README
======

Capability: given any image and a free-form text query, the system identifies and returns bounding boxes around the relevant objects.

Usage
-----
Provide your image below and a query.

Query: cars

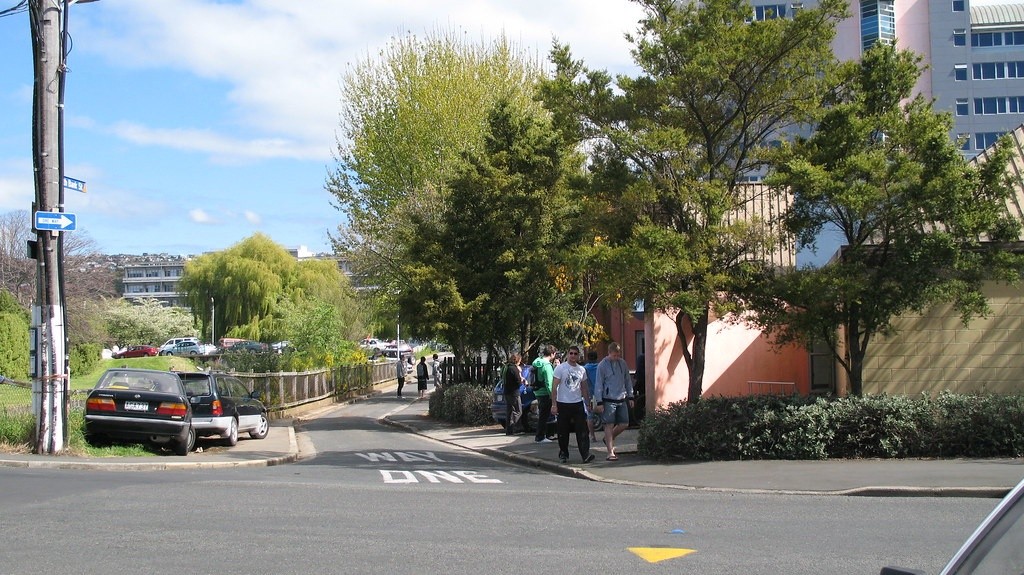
[491,364,640,433]
[111,344,158,358]
[84,368,196,456]
[356,337,453,373]
[880,479,1024,575]
[166,341,204,356]
[200,338,296,355]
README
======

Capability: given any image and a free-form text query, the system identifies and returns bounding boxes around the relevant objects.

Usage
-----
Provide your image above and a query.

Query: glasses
[570,352,578,356]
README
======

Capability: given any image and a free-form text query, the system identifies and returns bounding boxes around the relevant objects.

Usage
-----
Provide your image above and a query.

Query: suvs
[172,369,269,446]
[158,336,201,356]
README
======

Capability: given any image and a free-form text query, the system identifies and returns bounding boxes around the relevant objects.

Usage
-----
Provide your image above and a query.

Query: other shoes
[582,453,595,463]
[397,396,406,400]
[506,432,519,436]
[548,435,558,440]
[590,435,597,443]
[560,456,567,464]
[534,437,552,443]
[417,396,426,400]
[517,431,526,435]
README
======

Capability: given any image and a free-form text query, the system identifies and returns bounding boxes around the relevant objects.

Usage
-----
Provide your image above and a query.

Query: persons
[500,342,634,463]
[417,356,429,399]
[396,355,406,398]
[432,354,441,390]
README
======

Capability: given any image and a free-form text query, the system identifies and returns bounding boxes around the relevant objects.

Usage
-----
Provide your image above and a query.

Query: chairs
[112,382,129,388]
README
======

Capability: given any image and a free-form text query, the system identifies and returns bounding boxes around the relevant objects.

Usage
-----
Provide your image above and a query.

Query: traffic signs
[34,211,77,232]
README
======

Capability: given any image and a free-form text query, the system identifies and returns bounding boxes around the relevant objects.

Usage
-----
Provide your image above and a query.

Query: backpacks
[528,361,554,391]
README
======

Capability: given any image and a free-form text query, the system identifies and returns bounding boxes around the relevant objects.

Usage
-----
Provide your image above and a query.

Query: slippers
[605,454,618,461]
[603,437,617,454]
